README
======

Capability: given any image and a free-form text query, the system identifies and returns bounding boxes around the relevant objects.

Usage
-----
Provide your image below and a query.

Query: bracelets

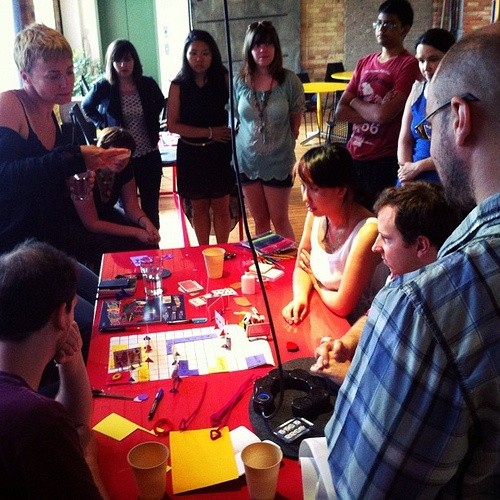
[207,125,217,140]
[137,214,148,224]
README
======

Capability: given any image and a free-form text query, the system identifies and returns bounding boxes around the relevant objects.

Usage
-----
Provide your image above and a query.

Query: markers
[148,389,163,419]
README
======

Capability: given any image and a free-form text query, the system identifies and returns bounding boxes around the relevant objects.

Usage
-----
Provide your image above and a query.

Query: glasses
[247,20,273,31]
[414,93,480,141]
[372,22,400,31]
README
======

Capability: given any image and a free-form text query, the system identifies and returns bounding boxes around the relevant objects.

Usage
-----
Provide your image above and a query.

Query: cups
[126,441,169,500]
[140,261,162,296]
[241,442,283,500]
[202,246,225,278]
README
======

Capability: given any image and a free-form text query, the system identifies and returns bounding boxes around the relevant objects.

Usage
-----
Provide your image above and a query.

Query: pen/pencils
[273,254,294,258]
[166,317,207,324]
[101,327,141,331]
[265,257,285,269]
[93,392,133,398]
[265,256,290,260]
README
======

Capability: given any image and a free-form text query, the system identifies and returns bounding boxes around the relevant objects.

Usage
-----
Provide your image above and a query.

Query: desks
[299,71,358,146]
[85,240,352,500]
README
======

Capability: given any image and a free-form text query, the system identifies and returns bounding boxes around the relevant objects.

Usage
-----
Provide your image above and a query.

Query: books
[98,294,187,330]
[98,277,136,297]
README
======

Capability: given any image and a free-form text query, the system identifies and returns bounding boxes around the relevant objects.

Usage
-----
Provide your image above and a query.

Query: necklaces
[250,74,276,133]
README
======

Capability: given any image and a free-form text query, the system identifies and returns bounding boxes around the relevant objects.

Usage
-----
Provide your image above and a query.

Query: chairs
[296,70,320,137]
[323,62,345,118]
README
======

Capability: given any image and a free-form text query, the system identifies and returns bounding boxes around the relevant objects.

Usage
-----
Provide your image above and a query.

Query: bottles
[241,268,255,295]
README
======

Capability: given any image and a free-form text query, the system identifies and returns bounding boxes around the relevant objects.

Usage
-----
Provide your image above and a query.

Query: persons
[82,38,168,232]
[0,238,92,500]
[394,27,456,188]
[309,178,457,378]
[279,144,388,327]
[224,21,306,240]
[329,0,417,166]
[0,23,129,331]
[323,23,500,499]
[68,127,161,254]
[165,29,233,247]
